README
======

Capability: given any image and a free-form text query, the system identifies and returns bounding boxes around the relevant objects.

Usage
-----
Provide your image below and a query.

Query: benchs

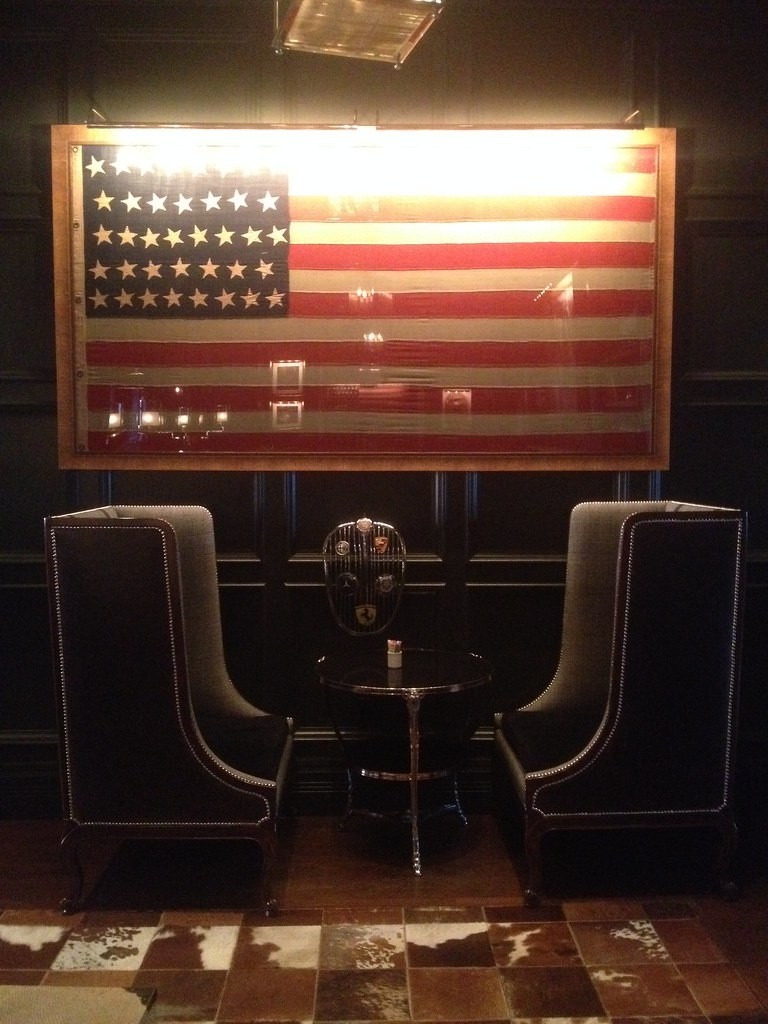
[43,502,294,917]
[484,498,749,907]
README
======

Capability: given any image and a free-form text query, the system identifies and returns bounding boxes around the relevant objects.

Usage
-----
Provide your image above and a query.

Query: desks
[317,650,492,878]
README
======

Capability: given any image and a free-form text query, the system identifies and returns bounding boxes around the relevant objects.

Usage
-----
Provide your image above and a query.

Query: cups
[387,651,402,668]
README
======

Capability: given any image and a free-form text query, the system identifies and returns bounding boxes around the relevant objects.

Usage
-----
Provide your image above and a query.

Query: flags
[69,144,655,460]
